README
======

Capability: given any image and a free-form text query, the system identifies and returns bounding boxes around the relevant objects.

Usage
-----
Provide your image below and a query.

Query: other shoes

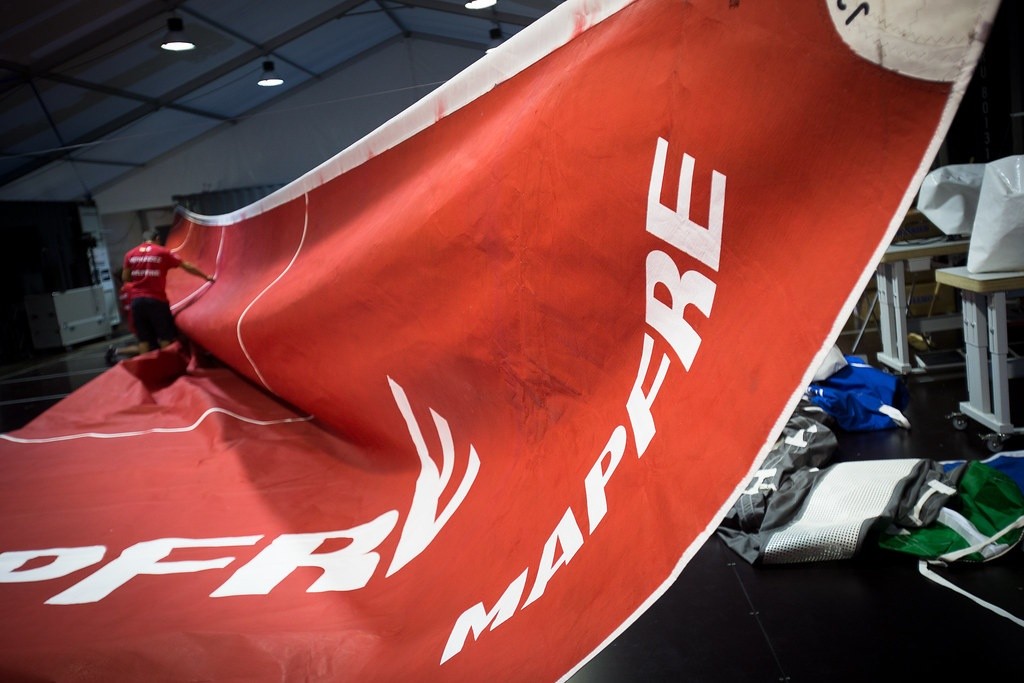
[105,344,116,368]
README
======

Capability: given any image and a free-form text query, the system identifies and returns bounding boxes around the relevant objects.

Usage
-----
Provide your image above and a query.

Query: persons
[106,231,216,366]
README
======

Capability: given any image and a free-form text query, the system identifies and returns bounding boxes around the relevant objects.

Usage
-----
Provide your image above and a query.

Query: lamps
[257,53,284,86]
[160,10,195,51]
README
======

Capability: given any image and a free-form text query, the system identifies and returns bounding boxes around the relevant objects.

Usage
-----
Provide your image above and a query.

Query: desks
[873,238,1024,452]
